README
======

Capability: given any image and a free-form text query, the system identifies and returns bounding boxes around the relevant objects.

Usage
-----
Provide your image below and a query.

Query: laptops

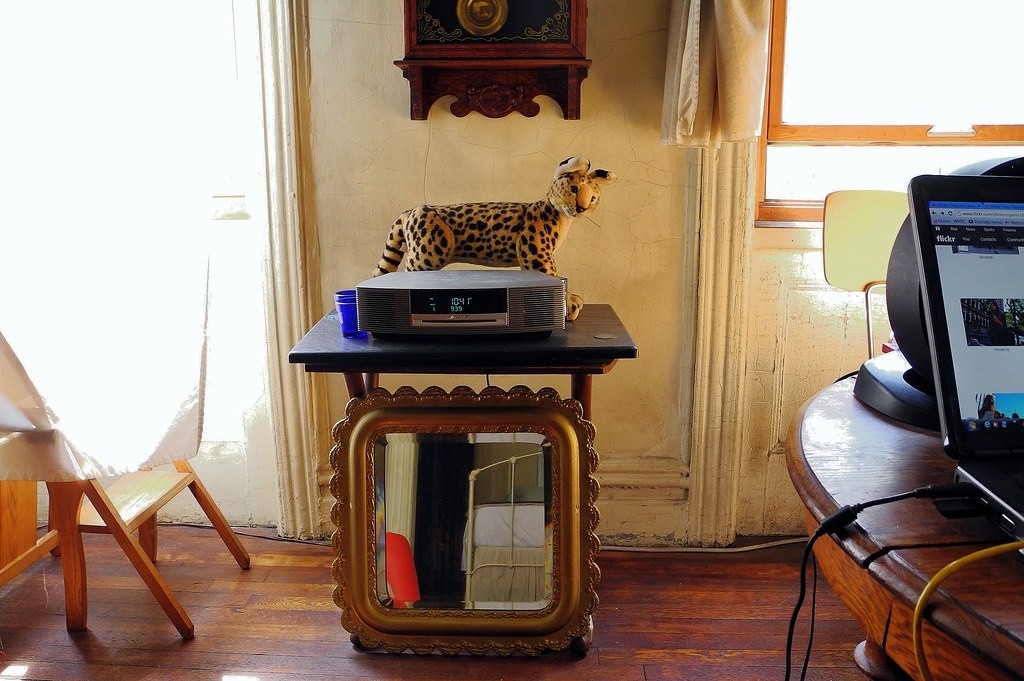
[909,175,1024,540]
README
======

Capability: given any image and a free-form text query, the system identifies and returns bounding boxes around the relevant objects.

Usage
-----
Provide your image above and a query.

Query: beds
[461,452,548,608]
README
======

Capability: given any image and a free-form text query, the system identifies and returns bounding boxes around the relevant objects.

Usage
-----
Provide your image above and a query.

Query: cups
[335,290,367,339]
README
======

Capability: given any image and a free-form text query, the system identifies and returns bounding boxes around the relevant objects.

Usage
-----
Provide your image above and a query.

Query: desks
[785,372,1024,680]
[289,302,637,656]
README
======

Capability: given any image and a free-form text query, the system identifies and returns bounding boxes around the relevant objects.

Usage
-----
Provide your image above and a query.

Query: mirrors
[328,386,600,654]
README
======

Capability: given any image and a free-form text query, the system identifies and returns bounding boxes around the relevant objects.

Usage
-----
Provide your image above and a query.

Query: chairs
[822,190,910,359]
